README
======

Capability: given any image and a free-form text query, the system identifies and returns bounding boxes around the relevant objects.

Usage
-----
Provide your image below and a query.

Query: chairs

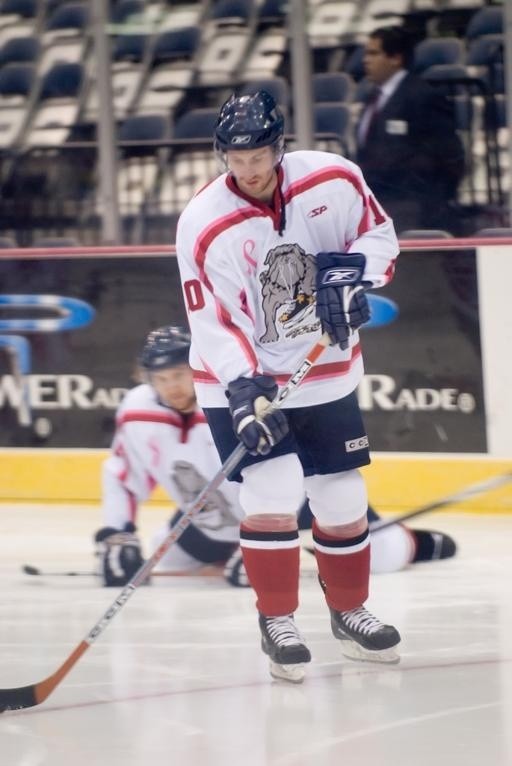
[0,0,511,247]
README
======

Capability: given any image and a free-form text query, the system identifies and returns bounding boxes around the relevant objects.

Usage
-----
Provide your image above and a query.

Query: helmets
[213,90,286,177]
[140,321,197,412]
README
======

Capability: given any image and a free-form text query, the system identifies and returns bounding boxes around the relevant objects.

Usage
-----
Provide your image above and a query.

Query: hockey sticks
[23,565,232,578]
[1,329,332,711]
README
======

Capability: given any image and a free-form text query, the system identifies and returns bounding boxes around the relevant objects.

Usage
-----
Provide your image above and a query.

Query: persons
[176,88,401,669]
[96,326,458,587]
[352,24,464,234]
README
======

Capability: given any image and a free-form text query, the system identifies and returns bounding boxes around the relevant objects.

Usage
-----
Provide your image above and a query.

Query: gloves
[95,523,146,590]
[222,373,292,460]
[313,252,372,350]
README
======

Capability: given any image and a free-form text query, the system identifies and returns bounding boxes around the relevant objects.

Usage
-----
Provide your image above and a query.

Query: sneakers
[408,527,459,564]
[330,603,403,652]
[258,613,313,668]
